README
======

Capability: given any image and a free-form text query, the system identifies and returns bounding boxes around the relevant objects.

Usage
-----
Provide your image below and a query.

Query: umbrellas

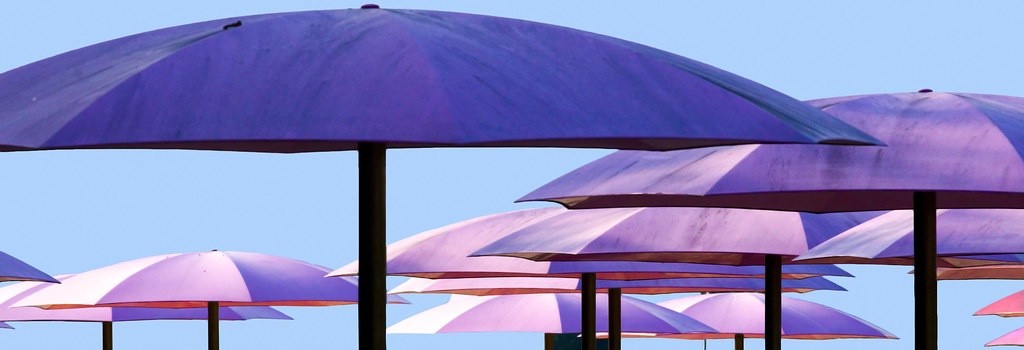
[0,5,1024,350]
